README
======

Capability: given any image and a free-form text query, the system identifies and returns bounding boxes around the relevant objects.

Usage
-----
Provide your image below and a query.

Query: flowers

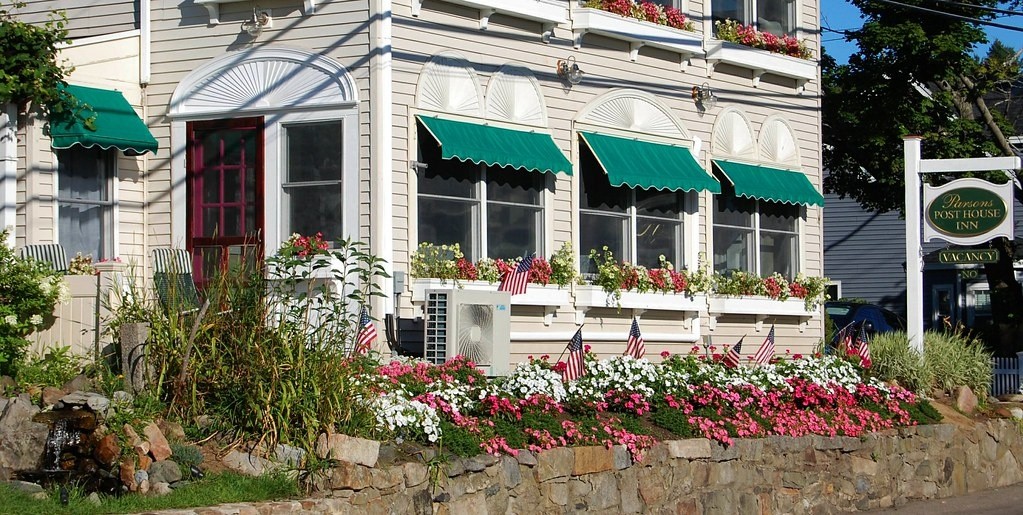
[279,230,330,258]
[65,252,123,276]
[588,247,710,314]
[712,268,831,311]
[410,240,576,288]
[584,0,696,32]
[714,17,813,61]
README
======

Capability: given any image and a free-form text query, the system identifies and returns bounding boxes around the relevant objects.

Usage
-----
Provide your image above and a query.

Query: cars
[824,302,906,355]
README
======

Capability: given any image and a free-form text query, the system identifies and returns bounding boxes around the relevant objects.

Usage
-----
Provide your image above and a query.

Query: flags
[755,325,775,366]
[562,328,586,384]
[354,307,377,354]
[840,323,853,349]
[854,326,872,366]
[627,319,645,359]
[723,337,743,369]
[500,255,532,296]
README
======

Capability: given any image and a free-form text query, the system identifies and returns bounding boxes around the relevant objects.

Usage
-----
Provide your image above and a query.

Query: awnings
[51,83,158,155]
[714,160,825,207]
[416,114,572,176]
[578,131,721,194]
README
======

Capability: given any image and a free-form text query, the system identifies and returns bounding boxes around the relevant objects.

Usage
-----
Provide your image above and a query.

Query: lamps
[557,55,585,84]
[692,82,719,110]
[246,4,272,37]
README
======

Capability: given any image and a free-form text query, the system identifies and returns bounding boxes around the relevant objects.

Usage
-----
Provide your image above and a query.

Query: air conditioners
[424,289,511,377]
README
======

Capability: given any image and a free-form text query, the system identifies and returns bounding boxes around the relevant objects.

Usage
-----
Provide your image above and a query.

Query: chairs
[151,248,233,329]
